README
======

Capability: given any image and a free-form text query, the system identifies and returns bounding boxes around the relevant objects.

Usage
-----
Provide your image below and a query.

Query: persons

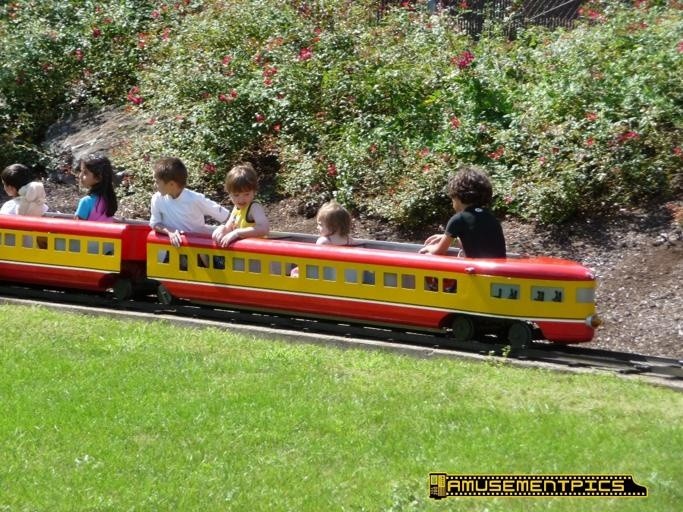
[148,155,232,270]
[212,164,269,272]
[74,155,118,223]
[0,164,49,218]
[290,200,360,283]
[418,165,507,293]
